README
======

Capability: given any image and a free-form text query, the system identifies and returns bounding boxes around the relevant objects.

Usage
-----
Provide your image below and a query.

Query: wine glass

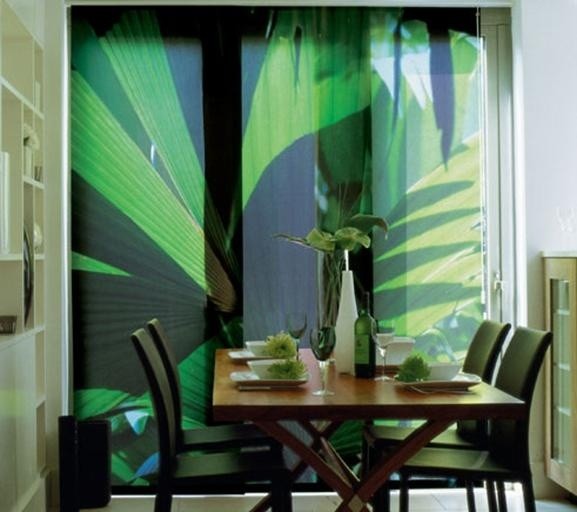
[285,310,308,362]
[309,326,336,396]
[371,317,396,383]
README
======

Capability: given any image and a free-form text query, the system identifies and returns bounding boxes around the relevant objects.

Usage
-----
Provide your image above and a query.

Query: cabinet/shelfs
[542,257,577,496]
[0,1,48,511]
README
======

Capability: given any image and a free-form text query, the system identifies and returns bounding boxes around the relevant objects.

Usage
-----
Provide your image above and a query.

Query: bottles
[352,290,377,380]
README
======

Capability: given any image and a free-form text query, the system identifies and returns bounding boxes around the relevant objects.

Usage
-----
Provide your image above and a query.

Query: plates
[227,350,278,362]
[392,372,483,387]
[374,354,418,372]
[232,370,309,386]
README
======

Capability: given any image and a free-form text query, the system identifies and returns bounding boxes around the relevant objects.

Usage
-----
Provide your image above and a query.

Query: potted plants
[271,207,390,327]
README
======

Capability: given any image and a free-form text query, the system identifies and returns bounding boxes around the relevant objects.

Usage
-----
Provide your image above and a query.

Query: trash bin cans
[79,420,111,507]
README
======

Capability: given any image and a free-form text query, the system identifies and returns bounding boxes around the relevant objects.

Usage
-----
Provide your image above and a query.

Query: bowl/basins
[246,358,290,377]
[243,340,271,354]
[422,359,462,379]
[376,335,416,354]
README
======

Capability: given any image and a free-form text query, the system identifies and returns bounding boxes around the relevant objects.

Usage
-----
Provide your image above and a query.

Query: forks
[409,386,476,395]
